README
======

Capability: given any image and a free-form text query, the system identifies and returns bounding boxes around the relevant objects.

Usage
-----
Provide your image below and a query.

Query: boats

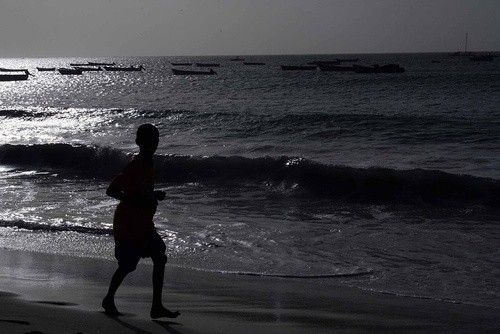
[468,52,493,63]
[281,64,317,72]
[171,67,218,77]
[243,62,265,66]
[232,57,245,62]
[308,58,405,77]
[171,62,193,66]
[0,57,144,86]
[196,62,221,68]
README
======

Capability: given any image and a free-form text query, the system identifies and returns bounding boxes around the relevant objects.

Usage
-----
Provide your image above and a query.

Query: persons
[101,124,181,319]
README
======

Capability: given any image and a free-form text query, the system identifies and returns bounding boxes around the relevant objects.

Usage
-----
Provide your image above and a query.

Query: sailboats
[449,33,474,57]
